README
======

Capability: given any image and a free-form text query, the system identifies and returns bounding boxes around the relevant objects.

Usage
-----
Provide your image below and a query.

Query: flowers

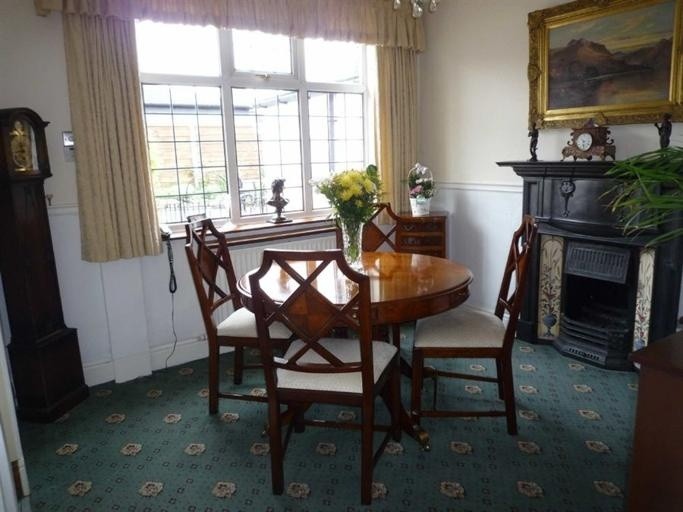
[405,163,438,201]
[310,163,384,219]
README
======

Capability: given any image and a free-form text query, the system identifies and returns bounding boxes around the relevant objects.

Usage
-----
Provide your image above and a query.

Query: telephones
[159,224,172,234]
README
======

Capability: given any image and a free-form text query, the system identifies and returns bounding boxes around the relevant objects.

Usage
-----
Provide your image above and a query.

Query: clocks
[0,109,90,425]
[561,129,615,160]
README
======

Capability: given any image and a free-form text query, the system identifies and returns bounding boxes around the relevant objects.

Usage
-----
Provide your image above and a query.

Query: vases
[410,195,430,215]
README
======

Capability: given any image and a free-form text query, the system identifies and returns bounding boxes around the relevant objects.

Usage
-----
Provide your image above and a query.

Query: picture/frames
[525,2,682,127]
